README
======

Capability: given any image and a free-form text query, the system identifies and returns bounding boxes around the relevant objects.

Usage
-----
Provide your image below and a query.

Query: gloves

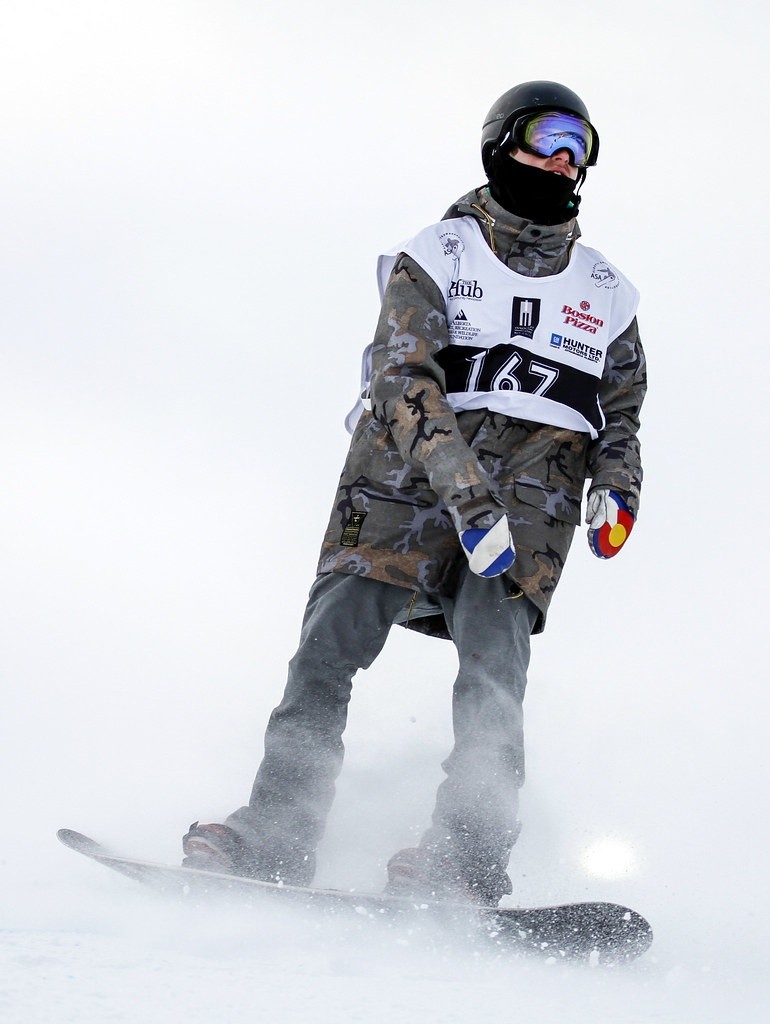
[459,513,516,578]
[585,489,635,560]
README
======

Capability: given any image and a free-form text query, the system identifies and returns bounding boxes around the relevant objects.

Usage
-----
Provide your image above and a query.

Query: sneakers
[183,820,316,888]
[382,848,502,908]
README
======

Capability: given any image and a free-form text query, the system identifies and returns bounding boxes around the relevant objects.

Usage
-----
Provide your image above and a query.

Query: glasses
[511,108,596,167]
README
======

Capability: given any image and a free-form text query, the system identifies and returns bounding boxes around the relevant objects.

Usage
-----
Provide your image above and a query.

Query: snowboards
[55,828,655,960]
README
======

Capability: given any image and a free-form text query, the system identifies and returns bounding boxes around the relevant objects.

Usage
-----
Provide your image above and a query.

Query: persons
[182,82,648,905]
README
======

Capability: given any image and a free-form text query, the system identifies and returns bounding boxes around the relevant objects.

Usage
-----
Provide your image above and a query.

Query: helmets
[481,81,600,177]
